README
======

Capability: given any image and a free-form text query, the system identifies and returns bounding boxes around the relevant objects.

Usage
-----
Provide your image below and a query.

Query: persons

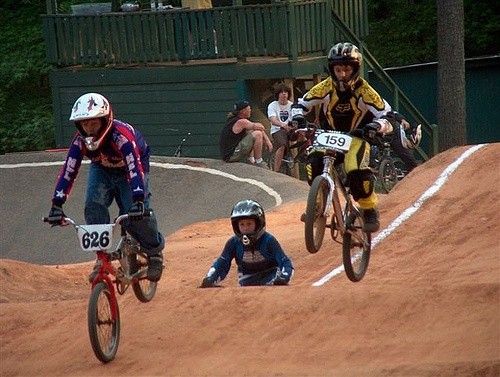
[268,84,299,180]
[202,199,293,286]
[48,92,165,282]
[377,110,417,174]
[288,41,396,233]
[219,99,274,169]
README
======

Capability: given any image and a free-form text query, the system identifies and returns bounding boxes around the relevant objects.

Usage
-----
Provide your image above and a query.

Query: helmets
[327,42,363,93]
[68,92,114,152]
[230,198,267,247]
[398,123,422,150]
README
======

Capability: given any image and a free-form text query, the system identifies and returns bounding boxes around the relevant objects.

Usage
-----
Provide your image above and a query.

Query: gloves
[201,277,214,287]
[291,114,306,129]
[268,277,287,285]
[363,121,381,138]
[124,194,146,222]
[48,199,69,225]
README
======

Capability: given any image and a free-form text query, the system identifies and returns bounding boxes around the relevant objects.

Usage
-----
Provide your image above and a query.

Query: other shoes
[147,252,164,282]
[361,208,379,233]
[248,159,256,165]
[255,161,270,170]
[300,202,320,223]
[89,259,110,282]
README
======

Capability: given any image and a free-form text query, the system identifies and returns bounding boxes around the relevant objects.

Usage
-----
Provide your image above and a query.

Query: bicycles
[42,207,164,363]
[289,127,371,283]
[172,113,412,194]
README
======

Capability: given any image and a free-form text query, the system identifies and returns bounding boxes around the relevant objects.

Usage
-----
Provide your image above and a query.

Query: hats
[233,101,249,111]
